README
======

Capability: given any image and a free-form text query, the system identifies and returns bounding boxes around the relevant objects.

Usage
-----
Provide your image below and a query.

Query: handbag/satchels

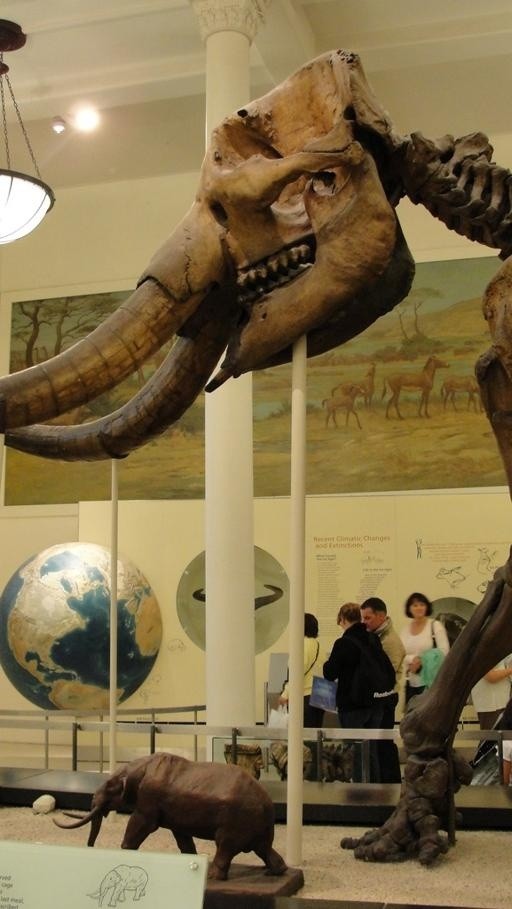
[309,676,338,714]
[266,709,289,729]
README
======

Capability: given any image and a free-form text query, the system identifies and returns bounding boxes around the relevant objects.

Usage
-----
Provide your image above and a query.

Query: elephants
[53,752,286,881]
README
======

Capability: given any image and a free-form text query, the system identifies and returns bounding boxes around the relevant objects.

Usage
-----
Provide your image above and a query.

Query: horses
[322,384,365,430]
[381,355,450,420]
[331,362,376,413]
[440,374,486,414]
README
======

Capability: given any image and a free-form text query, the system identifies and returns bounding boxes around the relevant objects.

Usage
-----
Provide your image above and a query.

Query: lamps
[0,19,54,246]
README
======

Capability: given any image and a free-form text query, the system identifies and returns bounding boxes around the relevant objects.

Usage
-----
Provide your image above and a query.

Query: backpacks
[343,635,399,706]
